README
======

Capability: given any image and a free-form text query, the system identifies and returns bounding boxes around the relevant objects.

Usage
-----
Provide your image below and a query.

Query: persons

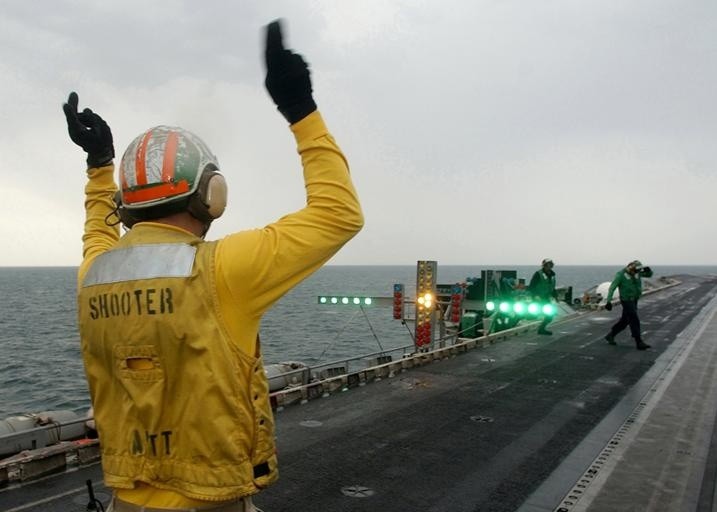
[600,260,655,350]
[508,275,516,286]
[527,258,559,335]
[59,16,365,511]
[501,274,514,295]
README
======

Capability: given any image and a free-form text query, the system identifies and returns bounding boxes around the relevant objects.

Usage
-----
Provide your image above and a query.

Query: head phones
[113,170,227,229]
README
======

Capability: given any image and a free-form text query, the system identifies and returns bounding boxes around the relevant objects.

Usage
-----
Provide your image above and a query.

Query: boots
[537,323,552,335]
[604,329,618,346]
[634,335,652,350]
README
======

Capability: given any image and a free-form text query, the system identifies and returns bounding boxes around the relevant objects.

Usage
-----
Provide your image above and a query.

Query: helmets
[116,123,223,210]
[541,257,554,265]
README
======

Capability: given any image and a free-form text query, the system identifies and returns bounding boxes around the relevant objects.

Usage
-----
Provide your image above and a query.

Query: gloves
[603,301,613,312]
[261,18,318,126]
[60,89,116,169]
